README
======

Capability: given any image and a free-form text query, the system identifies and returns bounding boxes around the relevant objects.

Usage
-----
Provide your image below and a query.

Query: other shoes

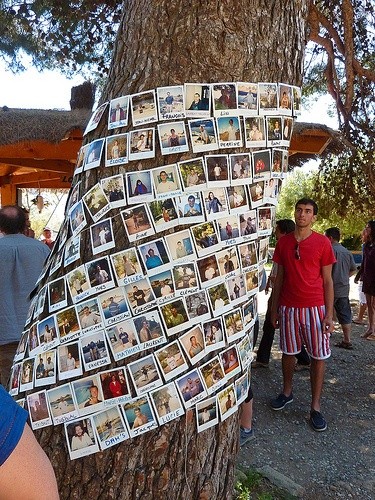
[366,334,375,340]
[294,364,311,371]
[251,361,269,368]
[352,319,364,324]
[360,331,373,338]
[239,426,254,446]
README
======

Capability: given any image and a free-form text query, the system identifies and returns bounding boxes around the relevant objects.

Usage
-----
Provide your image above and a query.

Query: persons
[270,198,338,432]
[239,269,267,446]
[352,220,375,341]
[12,80,302,452]
[1,204,52,390]
[0,385,61,500]
[324,227,357,350]
[252,219,311,371]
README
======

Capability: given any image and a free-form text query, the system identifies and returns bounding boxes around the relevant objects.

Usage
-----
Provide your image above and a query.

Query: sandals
[335,340,354,349]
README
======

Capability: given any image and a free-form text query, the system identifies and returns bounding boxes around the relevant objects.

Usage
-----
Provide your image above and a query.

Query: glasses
[295,245,301,260]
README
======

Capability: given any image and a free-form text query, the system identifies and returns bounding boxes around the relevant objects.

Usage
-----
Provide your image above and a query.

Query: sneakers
[310,408,327,432]
[270,392,293,411]
